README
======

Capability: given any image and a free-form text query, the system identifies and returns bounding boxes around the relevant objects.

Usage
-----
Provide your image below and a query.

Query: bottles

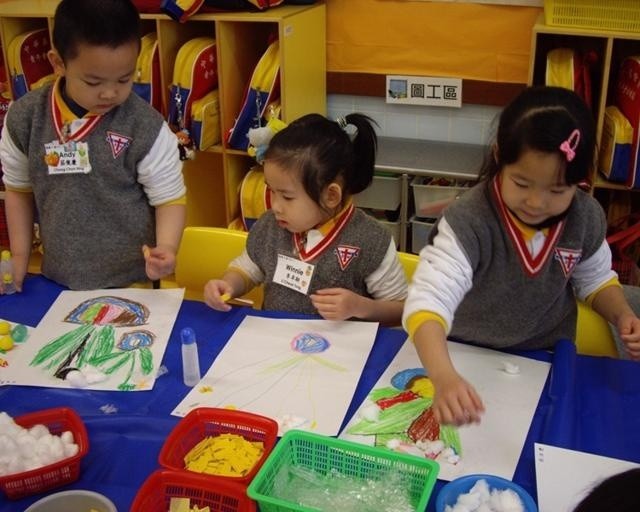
[180,327,202,387]
[0,250,17,295]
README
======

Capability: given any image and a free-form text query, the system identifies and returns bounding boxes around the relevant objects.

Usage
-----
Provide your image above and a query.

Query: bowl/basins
[24,489,119,511]
[435,473,536,511]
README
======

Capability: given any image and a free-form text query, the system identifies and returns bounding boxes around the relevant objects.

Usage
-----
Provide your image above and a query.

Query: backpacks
[599,57,640,189]
[168,38,220,161]
[240,166,271,231]
[225,40,280,151]
[6,28,56,101]
[546,48,597,112]
[132,33,164,117]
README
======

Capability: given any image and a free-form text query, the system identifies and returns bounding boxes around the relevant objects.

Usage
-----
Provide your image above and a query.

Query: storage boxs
[351,173,402,211]
[409,175,482,219]
[367,214,401,250]
[409,216,438,255]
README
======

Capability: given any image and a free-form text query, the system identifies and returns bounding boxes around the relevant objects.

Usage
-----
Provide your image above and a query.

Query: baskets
[0,406,90,499]
[158,407,278,483]
[247,430,440,512]
[129,470,256,511]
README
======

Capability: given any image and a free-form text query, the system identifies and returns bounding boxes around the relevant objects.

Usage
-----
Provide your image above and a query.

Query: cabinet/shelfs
[526,9,638,287]
[328,132,498,257]
[0,1,328,234]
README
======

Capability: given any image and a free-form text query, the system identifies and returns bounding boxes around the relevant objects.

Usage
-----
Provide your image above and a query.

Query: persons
[203,112,408,321]
[0,0,189,296]
[401,85,640,428]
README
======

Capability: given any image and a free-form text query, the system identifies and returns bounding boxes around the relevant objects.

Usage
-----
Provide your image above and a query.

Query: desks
[1,270,640,512]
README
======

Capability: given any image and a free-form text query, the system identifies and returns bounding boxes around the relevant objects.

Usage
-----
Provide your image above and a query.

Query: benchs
[175,226,622,360]
